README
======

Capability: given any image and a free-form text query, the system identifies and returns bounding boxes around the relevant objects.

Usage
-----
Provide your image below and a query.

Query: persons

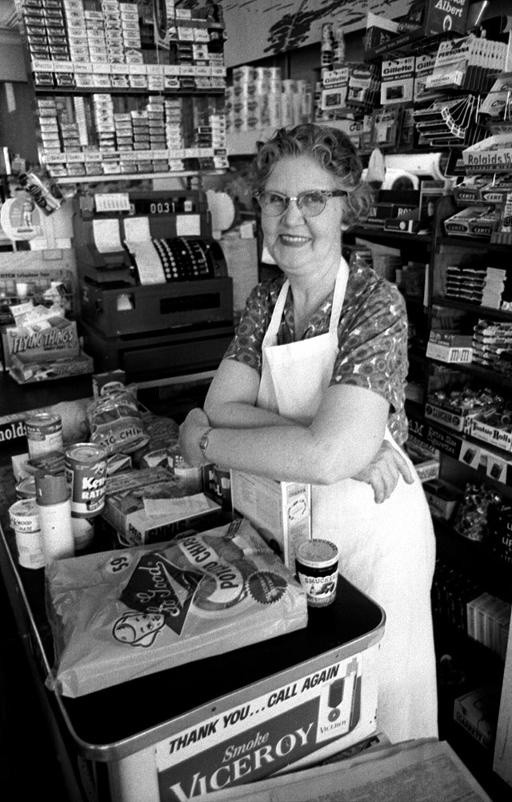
[178,122,443,747]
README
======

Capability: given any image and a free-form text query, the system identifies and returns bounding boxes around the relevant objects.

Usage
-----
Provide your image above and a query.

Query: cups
[249,186,352,218]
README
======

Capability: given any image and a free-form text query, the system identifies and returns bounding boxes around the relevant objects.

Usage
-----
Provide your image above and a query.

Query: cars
[17,282,35,305]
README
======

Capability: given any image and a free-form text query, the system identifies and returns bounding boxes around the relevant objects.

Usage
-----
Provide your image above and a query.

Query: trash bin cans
[199,427,219,467]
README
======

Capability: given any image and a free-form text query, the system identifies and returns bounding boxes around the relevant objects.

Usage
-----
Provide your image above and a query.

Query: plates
[347,188,512,736]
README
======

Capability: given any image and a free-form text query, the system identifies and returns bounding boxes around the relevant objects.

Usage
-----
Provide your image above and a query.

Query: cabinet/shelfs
[34,472,77,566]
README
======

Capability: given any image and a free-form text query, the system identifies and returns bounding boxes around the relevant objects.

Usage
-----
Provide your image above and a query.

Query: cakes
[295,539,339,609]
[25,412,64,472]
[65,443,107,519]
[224,65,307,128]
